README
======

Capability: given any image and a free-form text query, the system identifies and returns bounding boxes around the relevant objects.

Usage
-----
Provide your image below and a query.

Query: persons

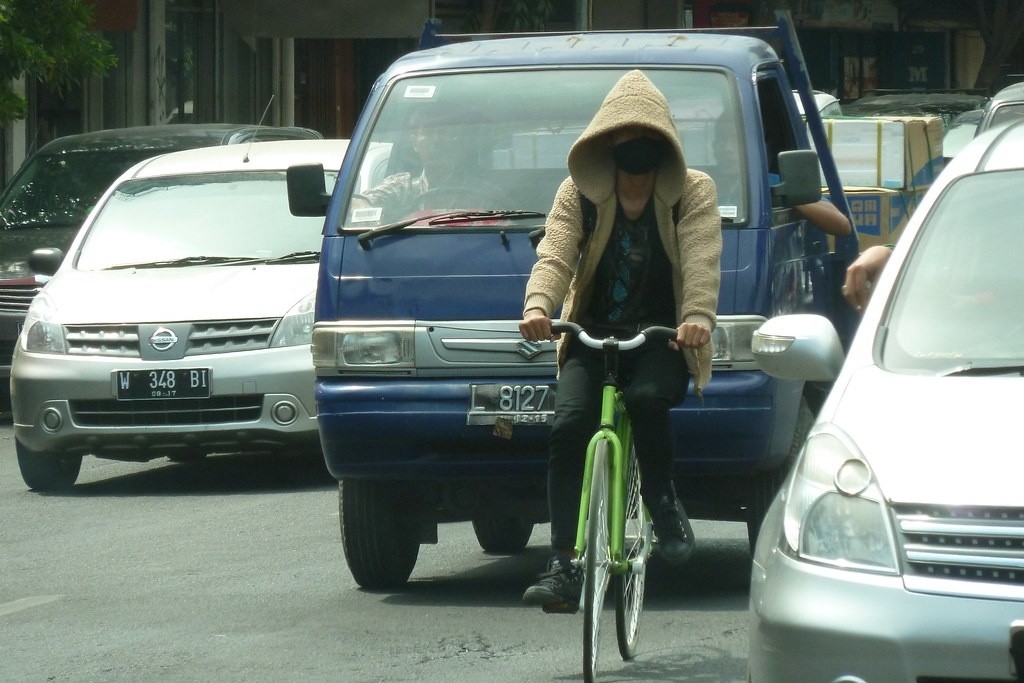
[356,99,521,225]
[517,68,723,608]
[842,243,897,316]
[708,113,852,236]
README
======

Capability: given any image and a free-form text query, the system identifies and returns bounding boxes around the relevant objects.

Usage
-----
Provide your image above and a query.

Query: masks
[612,137,663,175]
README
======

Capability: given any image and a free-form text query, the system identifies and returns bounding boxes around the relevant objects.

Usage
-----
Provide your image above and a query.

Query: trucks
[285,6,866,589]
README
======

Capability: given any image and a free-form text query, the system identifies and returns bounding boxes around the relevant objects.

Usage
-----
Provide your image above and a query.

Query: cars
[1,125,326,428]
[9,138,397,491]
[743,112,1024,683]
[785,81,1024,163]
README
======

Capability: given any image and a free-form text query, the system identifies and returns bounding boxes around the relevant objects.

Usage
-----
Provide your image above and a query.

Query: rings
[848,295,883,297]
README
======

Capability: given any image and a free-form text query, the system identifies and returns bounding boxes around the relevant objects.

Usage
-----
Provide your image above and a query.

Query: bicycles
[541,320,703,683]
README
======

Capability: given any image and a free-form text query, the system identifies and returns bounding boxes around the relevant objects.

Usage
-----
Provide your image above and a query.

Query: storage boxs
[804,116,946,254]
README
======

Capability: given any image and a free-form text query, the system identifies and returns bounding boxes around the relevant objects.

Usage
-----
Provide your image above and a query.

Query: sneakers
[522,552,584,611]
[642,487,695,567]
